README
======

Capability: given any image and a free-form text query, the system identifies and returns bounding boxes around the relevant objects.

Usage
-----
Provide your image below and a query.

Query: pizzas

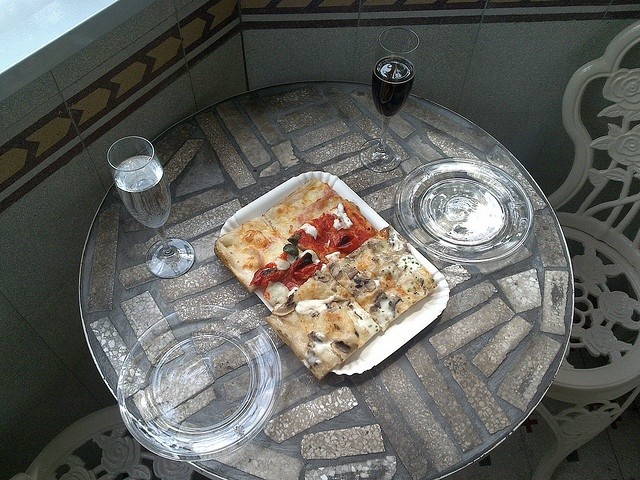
[214,177,378,311]
[265,271,379,382]
[329,224,438,333]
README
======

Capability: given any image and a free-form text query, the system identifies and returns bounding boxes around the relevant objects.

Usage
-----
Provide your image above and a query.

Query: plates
[116,305,283,463]
[218,171,450,376]
[395,157,535,263]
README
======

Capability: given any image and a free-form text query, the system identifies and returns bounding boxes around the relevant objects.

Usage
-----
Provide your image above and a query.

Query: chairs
[530,20,640,480]
[9,404,202,480]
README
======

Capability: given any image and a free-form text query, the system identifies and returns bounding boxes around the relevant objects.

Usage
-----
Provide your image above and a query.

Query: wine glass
[359,27,420,174]
[107,135,196,279]
